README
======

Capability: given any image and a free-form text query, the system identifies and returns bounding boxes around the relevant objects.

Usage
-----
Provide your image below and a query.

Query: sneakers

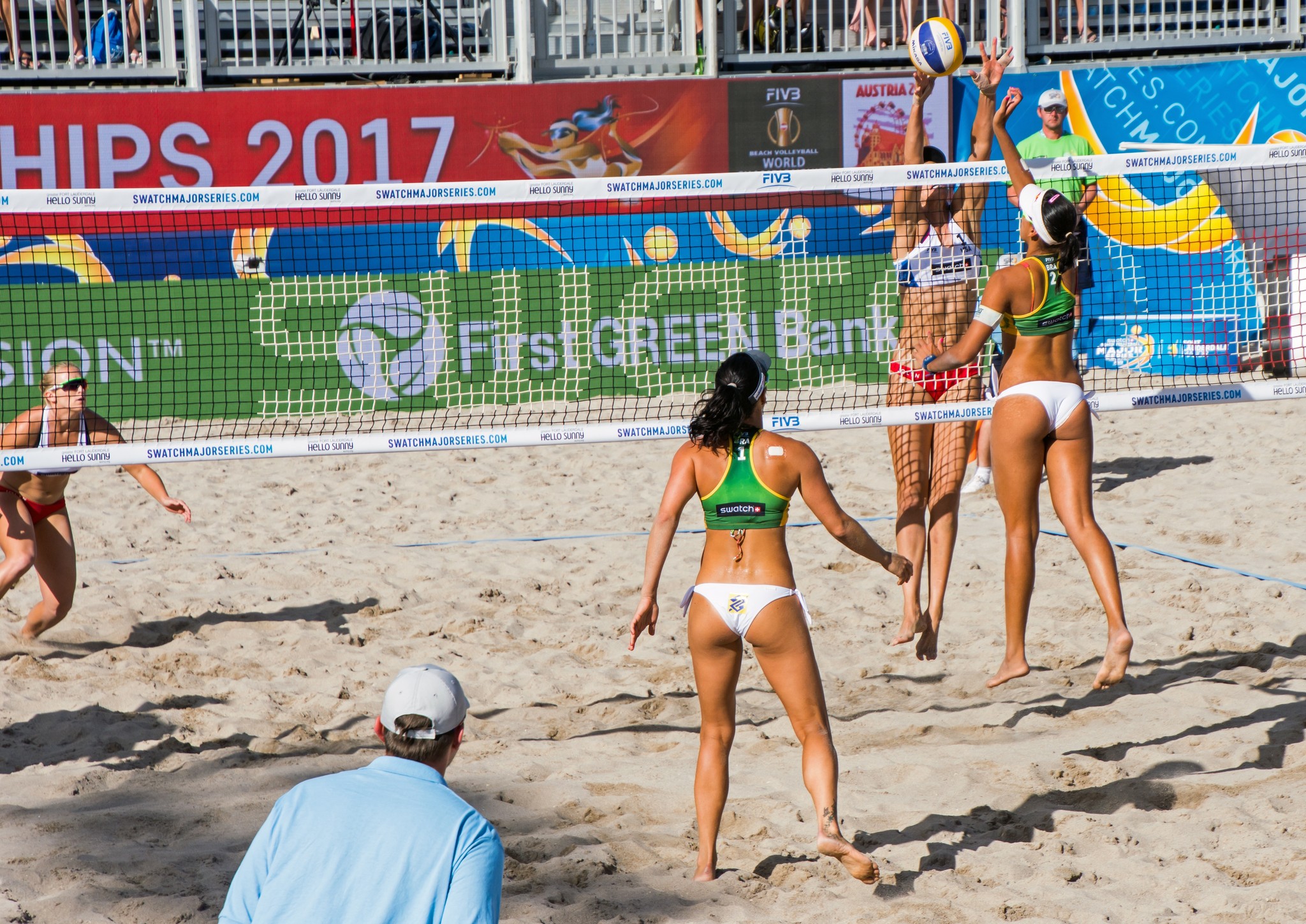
[740,29,763,50]
[693,36,703,74]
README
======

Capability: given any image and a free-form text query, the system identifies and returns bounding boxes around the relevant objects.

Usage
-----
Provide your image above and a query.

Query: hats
[380,664,471,742]
[923,145,947,163]
[1039,88,1068,108]
[1019,183,1077,246]
[731,349,772,403]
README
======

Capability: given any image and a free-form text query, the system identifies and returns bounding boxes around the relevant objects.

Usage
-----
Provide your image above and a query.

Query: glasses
[47,377,88,391]
[1040,105,1065,113]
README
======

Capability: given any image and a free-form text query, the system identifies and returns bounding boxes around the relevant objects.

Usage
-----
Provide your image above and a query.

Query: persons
[886,38,1014,654]
[218,663,503,924]
[0,0,1097,87]
[628,350,914,883]
[0,361,192,638]
[960,88,1105,495]
[912,77,1132,692]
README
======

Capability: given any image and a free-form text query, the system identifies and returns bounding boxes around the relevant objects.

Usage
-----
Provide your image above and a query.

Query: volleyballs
[907,17,967,77]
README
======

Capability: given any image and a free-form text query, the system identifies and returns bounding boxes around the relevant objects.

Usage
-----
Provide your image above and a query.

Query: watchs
[923,355,940,375]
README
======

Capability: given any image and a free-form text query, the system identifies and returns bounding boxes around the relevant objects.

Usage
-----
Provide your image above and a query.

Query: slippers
[1079,28,1097,42]
[128,52,152,64]
[11,56,41,68]
[1047,30,1068,41]
[888,37,904,45]
[66,53,85,64]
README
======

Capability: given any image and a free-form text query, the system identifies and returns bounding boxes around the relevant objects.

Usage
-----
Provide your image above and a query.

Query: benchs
[0,0,1306,85]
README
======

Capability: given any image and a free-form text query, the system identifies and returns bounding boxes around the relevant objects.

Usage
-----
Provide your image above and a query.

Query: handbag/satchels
[756,5,826,52]
[84,9,124,63]
